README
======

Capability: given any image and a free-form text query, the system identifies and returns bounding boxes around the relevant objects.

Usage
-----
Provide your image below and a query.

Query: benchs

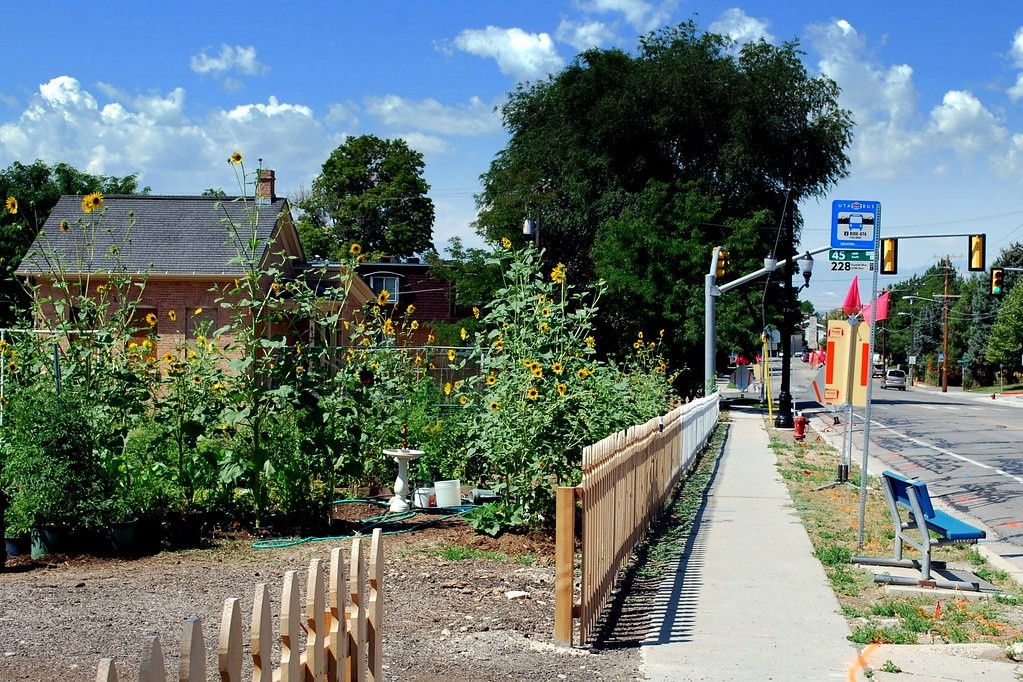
[879,471,987,581]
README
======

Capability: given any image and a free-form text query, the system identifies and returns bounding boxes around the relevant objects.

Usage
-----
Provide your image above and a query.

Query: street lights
[897,312,914,386]
[764,185,815,431]
[901,295,948,393]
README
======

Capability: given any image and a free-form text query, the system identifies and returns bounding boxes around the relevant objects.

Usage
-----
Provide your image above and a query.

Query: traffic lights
[990,267,1005,298]
[713,246,731,278]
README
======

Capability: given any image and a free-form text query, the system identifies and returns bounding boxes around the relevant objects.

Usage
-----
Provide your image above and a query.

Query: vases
[3,494,330,563]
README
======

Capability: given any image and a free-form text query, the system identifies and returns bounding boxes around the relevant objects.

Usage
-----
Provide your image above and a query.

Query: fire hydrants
[793,416,808,442]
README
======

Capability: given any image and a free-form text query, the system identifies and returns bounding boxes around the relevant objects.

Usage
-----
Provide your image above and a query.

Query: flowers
[1,148,285,509]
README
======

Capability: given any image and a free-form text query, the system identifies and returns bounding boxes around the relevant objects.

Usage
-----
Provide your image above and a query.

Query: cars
[880,369,907,390]
[794,350,811,364]
[872,361,887,378]
